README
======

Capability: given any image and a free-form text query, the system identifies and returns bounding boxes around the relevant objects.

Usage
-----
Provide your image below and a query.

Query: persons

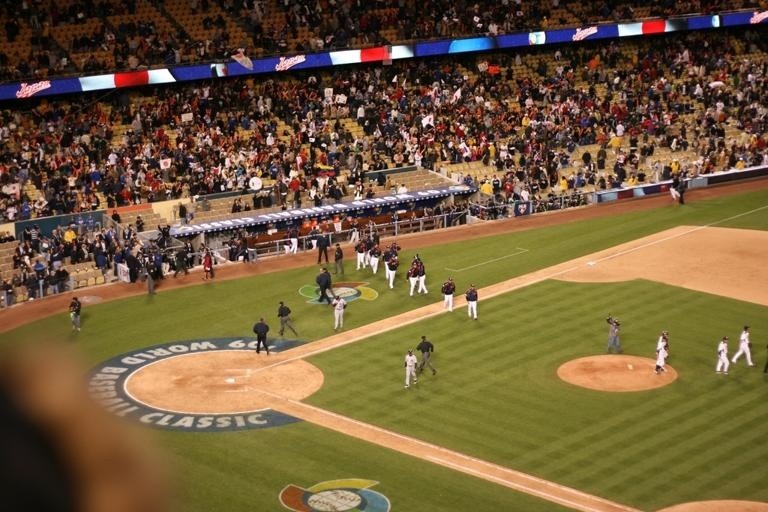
[654,343,669,374]
[0,1,765,304]
[403,348,419,389]
[441,277,456,314]
[607,314,625,355]
[69,297,83,331]
[731,324,756,368]
[464,283,478,321]
[277,301,299,337]
[714,335,730,375]
[416,335,438,375]
[656,330,670,358]
[252,317,269,351]
[332,295,347,329]
